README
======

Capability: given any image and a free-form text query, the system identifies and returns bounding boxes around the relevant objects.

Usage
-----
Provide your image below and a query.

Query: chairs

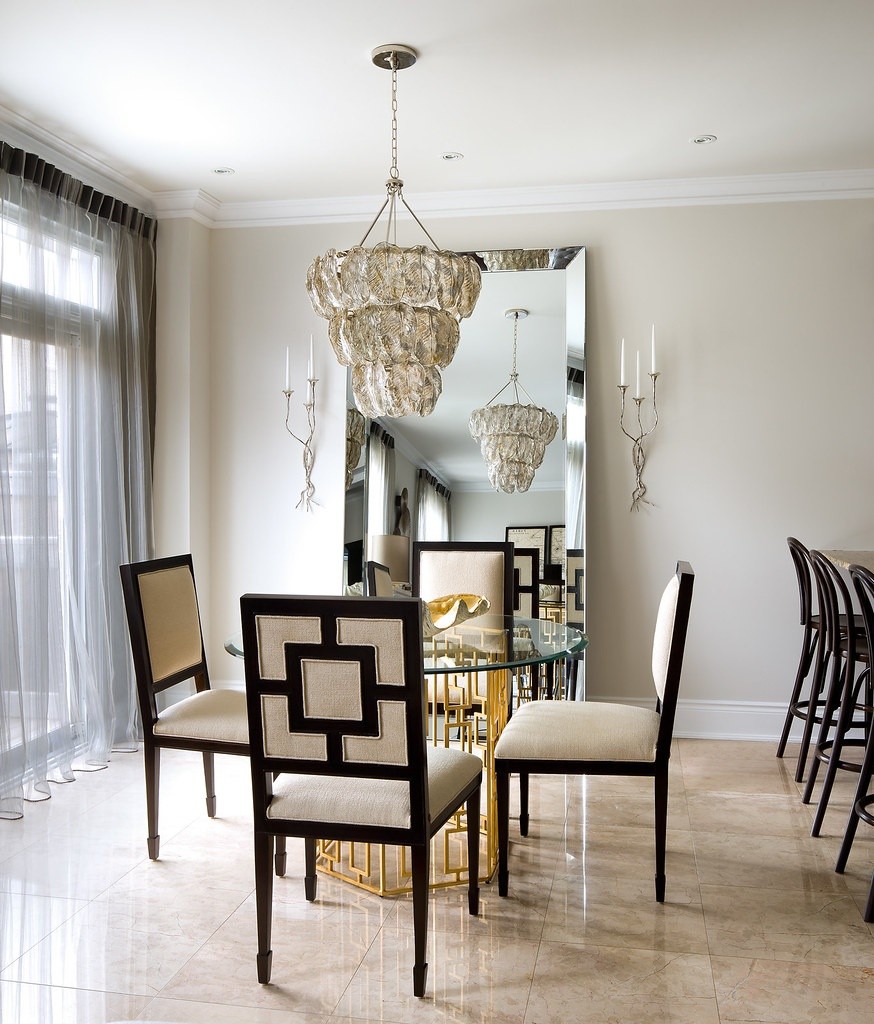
[412,539,515,744]
[236,589,484,999]
[119,553,289,879]
[773,534,874,924]
[537,580,562,604]
[513,547,541,620]
[491,559,696,902]
[367,561,395,599]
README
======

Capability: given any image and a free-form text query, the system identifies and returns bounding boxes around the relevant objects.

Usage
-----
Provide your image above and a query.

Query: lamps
[468,307,560,496]
[303,44,485,423]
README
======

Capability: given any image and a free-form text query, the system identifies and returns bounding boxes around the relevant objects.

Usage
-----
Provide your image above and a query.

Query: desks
[538,604,566,623]
[818,548,874,576]
[221,612,590,902]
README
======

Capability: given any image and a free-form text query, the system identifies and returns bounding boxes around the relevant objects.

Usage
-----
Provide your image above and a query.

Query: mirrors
[338,244,590,702]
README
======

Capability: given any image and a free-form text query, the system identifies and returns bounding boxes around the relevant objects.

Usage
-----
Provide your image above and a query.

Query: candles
[637,349,640,397]
[310,333,315,378]
[286,346,291,390]
[651,323,656,374]
[620,338,625,385]
[307,360,311,402]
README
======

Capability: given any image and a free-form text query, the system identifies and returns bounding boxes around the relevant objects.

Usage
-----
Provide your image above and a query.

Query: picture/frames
[548,524,567,584]
[506,526,547,583]
